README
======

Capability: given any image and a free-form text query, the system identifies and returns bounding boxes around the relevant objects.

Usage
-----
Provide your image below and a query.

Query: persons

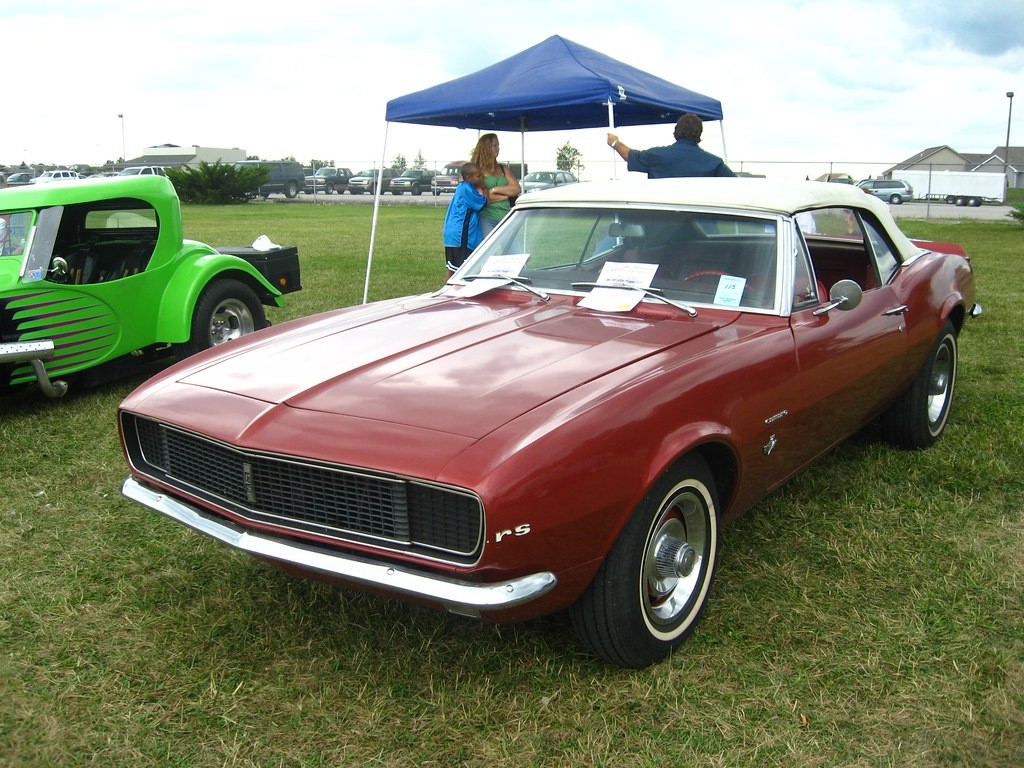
[472,133,521,265]
[608,114,737,235]
[444,163,489,286]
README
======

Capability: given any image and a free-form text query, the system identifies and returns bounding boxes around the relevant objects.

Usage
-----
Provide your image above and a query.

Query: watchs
[611,139,619,147]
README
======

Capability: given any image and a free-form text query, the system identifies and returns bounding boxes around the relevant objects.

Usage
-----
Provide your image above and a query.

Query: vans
[0,165,174,184]
[227,160,306,198]
[857,180,913,205]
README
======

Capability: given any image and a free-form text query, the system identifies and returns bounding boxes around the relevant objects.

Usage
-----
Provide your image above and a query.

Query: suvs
[347,168,400,196]
[302,168,354,196]
[389,168,442,196]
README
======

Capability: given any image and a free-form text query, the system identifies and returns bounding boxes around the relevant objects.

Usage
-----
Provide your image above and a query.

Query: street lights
[1003,92,1014,172]
[118,114,125,160]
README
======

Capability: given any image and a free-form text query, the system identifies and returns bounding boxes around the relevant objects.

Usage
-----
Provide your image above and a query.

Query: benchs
[658,240,878,302]
[60,239,158,285]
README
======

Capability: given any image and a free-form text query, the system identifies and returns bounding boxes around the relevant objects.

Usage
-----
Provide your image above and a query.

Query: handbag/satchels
[499,163,518,207]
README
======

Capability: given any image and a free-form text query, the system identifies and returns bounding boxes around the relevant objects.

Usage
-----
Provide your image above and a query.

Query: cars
[119,178,983,672]
[517,171,580,195]
[0,180,303,400]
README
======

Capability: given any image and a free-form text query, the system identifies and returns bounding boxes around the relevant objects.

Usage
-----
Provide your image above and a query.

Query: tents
[362,34,733,303]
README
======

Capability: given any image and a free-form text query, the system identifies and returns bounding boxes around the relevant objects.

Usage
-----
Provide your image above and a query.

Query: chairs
[744,271,827,305]
[600,227,682,281]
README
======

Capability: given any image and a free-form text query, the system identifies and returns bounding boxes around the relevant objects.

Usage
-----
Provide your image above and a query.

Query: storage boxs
[219,246,303,294]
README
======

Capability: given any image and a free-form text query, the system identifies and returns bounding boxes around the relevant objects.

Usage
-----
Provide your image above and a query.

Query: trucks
[855,170,1007,208]
[430,160,528,196]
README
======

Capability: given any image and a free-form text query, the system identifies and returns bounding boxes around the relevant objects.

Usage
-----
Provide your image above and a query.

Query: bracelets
[493,186,494,194]
